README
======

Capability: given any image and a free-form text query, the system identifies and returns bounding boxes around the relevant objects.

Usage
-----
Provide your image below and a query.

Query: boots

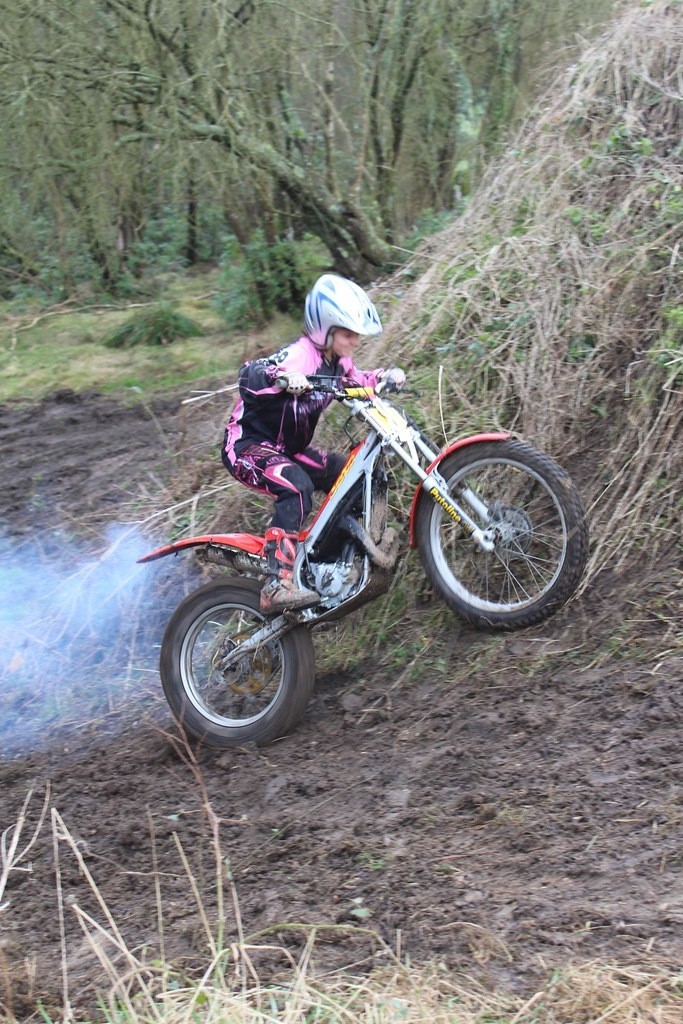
[259,527,321,614]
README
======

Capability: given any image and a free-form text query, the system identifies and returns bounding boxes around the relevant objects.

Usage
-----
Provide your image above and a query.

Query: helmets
[304,274,382,349]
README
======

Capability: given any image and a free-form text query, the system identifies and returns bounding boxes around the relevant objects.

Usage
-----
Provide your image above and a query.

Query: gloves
[378,368,406,395]
[276,370,308,396]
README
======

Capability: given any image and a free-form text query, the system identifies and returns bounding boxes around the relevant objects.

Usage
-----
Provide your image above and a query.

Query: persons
[221,273,408,617]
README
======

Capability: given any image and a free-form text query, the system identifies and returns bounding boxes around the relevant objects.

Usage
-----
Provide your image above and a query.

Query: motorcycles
[155,367,591,751]
